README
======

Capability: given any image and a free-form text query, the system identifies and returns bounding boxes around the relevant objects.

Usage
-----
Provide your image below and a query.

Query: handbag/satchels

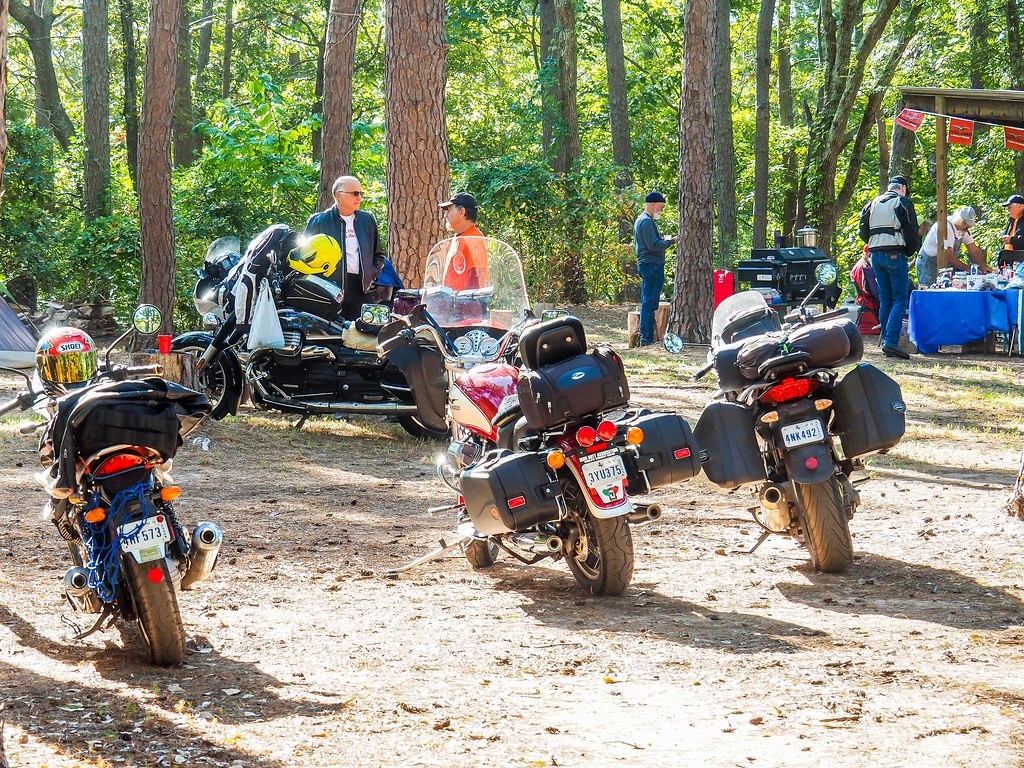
[247,277,285,349]
[516,348,630,428]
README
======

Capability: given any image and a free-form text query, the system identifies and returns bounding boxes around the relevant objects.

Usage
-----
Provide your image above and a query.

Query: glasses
[334,190,364,197]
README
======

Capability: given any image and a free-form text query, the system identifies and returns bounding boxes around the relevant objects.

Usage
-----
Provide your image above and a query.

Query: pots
[795,225,819,248]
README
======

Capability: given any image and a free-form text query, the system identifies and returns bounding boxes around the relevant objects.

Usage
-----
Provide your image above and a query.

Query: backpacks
[220,224,290,294]
[72,398,183,457]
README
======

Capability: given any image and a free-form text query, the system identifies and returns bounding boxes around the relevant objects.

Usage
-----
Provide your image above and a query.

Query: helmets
[36,327,98,399]
[286,233,342,278]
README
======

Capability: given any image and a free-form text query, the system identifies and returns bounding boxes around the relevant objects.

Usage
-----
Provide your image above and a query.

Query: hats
[438,192,479,210]
[864,244,869,252]
[645,192,664,202]
[1001,195,1024,207]
[958,204,979,227]
[916,216,925,226]
[889,175,909,196]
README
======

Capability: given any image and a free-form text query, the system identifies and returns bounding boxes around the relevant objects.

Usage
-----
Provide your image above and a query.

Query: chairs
[851,280,882,331]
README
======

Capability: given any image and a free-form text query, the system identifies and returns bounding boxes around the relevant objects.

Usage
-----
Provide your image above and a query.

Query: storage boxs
[835,363,905,458]
[80,405,177,464]
[607,407,702,495]
[693,402,767,487]
[282,271,346,321]
[460,448,568,535]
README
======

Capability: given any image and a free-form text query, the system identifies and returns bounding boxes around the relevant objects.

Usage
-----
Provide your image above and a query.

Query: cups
[664,235,671,241]
[1003,235,1009,243]
[158,335,172,354]
[781,237,787,248]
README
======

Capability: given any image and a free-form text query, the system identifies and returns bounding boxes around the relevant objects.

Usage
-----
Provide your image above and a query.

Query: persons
[633,191,678,347]
[300,174,385,321]
[370,257,404,312]
[916,205,996,289]
[850,243,879,316]
[999,194,1024,250]
[438,191,489,325]
[908,215,933,269]
[859,175,918,360]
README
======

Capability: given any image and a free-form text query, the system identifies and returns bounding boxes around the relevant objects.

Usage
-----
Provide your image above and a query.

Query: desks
[907,290,1020,355]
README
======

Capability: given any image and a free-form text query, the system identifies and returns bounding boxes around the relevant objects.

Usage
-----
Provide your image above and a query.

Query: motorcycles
[376,237,701,598]
[662,264,906,573]
[0,304,222,666]
[169,222,522,441]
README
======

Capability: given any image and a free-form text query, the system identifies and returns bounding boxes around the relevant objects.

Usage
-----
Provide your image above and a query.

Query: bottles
[760,289,779,305]
[993,262,1020,289]
[775,230,780,248]
[840,301,858,324]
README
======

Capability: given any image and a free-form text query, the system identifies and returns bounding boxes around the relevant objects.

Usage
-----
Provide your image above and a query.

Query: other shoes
[640,339,660,346]
[335,411,349,420]
[880,344,910,359]
[387,414,399,422]
